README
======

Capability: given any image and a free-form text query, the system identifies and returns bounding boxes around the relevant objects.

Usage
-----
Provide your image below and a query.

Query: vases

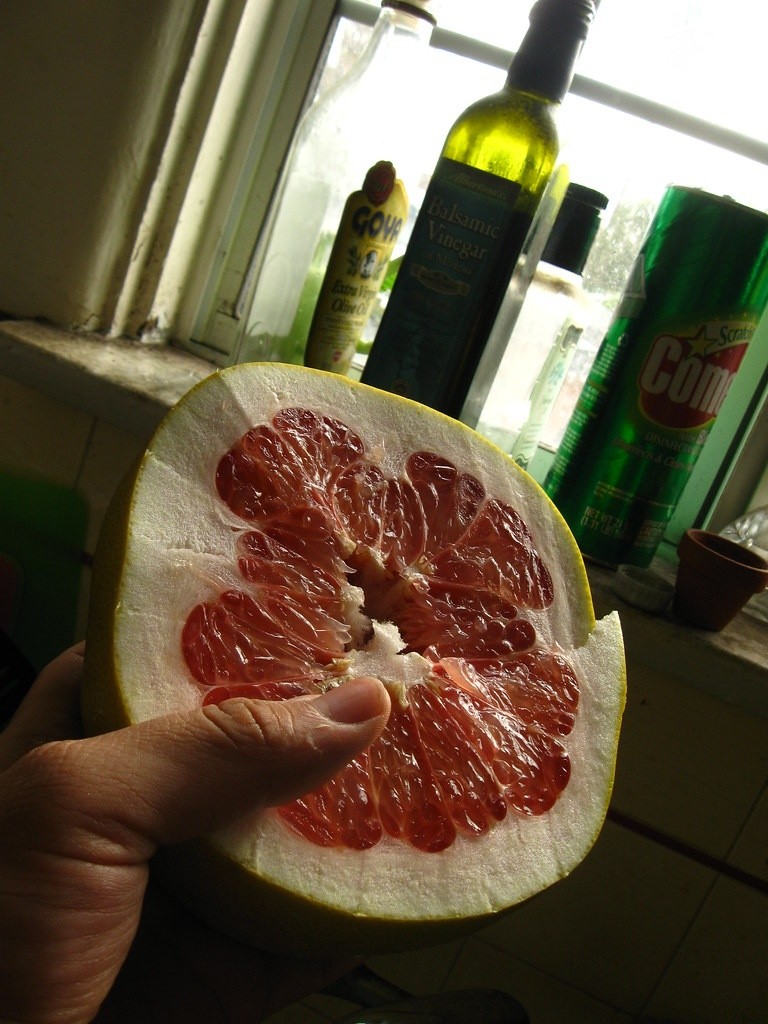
[674,528,768,631]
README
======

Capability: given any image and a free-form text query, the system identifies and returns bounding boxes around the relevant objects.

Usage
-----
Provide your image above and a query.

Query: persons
[0,639,392,1024]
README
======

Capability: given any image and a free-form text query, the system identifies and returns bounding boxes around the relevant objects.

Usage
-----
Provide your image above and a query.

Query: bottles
[359,0,603,428]
[235,1,438,378]
[462,181,610,473]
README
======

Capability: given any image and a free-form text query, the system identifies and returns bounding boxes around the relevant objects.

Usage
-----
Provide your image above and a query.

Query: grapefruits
[85,363,628,937]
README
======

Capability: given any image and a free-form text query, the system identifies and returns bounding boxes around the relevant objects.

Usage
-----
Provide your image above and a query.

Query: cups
[675,529,768,632]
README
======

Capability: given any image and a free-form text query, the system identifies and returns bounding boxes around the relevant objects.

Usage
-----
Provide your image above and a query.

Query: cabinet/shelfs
[2,373,768,1023]
